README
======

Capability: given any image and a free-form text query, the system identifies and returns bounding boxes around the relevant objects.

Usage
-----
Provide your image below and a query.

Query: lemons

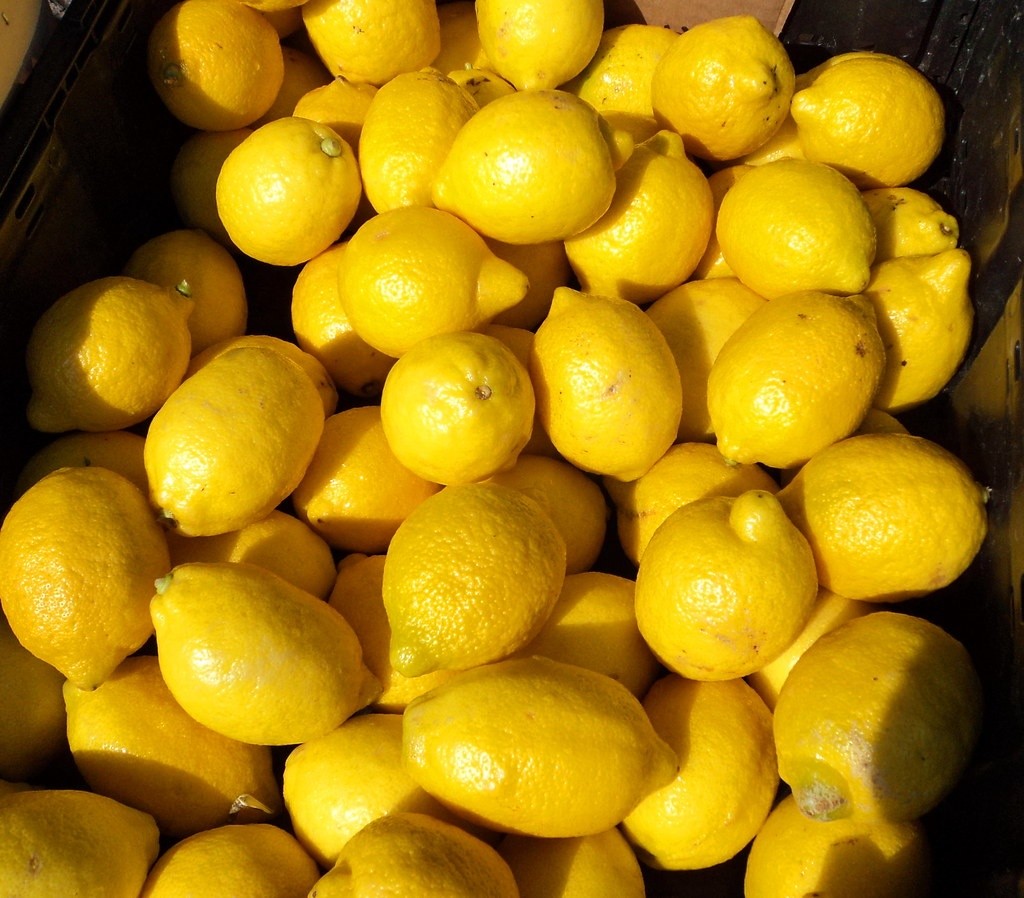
[0,0,991,898]
